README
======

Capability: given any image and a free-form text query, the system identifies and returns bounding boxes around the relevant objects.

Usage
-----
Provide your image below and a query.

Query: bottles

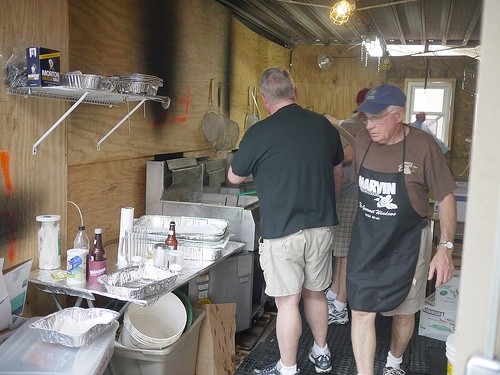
[165,221,178,251]
[88,228,107,285]
[35,215,62,270]
[73,225,91,280]
[167,251,189,272]
[124,226,146,267]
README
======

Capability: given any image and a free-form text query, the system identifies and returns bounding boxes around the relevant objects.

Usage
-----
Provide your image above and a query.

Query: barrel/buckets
[117,294,188,352]
[445,330,491,375]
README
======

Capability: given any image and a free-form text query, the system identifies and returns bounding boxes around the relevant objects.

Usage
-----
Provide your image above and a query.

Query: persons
[227,68,457,375]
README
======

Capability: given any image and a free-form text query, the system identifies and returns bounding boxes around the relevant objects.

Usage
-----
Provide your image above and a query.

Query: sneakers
[251,363,301,375]
[383,366,406,375]
[324,287,350,325]
[308,345,332,374]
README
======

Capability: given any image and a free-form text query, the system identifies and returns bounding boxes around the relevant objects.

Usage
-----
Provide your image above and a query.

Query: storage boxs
[0,317,119,374]
[197,301,236,375]
[433,269,460,310]
[105,308,205,375]
[418,290,456,340]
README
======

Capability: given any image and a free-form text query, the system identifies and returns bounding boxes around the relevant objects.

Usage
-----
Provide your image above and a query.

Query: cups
[153,243,171,270]
[66,248,88,285]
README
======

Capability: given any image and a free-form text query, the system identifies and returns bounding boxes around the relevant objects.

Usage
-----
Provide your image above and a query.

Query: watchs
[438,241,455,251]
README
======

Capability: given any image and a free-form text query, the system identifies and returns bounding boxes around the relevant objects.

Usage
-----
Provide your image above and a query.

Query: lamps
[363,33,384,58]
[329,0,352,25]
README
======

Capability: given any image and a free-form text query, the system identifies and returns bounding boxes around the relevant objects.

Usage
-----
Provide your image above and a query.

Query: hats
[356,87,369,102]
[353,84,407,114]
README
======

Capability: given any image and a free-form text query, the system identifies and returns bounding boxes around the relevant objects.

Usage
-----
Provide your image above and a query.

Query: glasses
[360,108,401,123]
